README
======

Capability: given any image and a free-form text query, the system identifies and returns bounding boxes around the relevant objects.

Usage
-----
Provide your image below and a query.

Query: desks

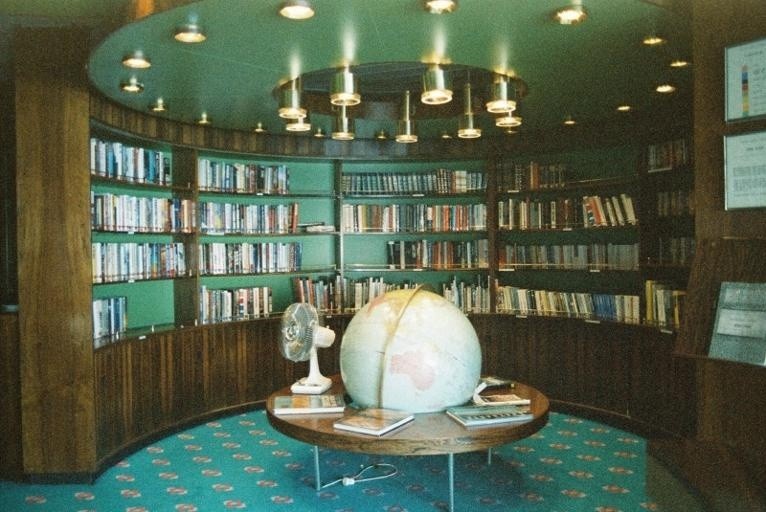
[265,372,552,510]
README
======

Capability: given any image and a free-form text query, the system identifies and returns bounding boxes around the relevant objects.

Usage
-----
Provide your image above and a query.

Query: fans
[278,300,338,395]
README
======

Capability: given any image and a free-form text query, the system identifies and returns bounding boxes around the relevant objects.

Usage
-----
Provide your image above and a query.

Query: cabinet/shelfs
[494,131,641,435]
[16,79,196,483]
[645,1,765,512]
[173,132,343,424]
[640,76,694,439]
[340,141,494,406]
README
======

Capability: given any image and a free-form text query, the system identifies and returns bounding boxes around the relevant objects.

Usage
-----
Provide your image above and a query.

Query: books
[92,137,195,340]
[341,168,491,314]
[332,408,415,436]
[724,43,766,122]
[481,375,516,391]
[273,393,347,415]
[722,131,766,211]
[495,163,641,326]
[708,279,766,368]
[445,403,533,429]
[475,392,529,405]
[646,138,694,319]
[197,160,341,325]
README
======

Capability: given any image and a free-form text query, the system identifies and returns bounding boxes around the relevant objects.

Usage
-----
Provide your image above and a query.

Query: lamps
[395,120,419,143]
[457,113,482,139]
[279,89,309,119]
[495,112,522,129]
[417,68,454,106]
[486,80,517,113]
[331,117,356,142]
[328,72,362,106]
[287,116,312,132]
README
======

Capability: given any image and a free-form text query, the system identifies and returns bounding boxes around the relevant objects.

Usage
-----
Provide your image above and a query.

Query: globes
[340,284,482,413]
